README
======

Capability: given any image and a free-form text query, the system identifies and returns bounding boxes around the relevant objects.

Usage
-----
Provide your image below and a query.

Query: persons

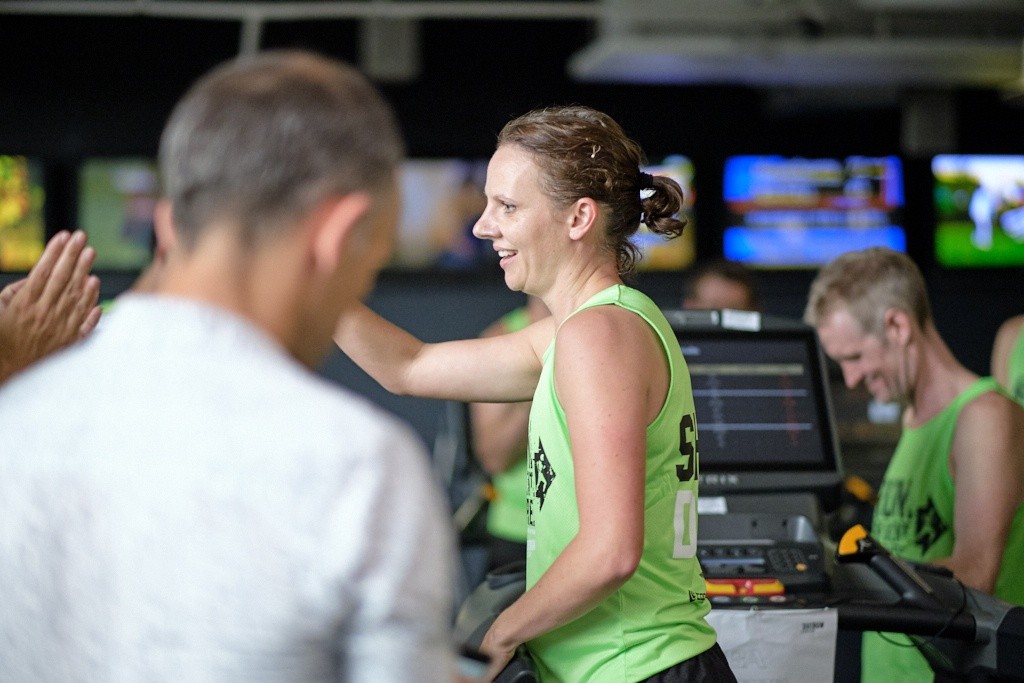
[0,229,102,385]
[332,106,736,683]
[0,48,473,683]
[990,314,1024,404]
[802,249,1024,683]
[470,293,553,574]
[681,262,754,310]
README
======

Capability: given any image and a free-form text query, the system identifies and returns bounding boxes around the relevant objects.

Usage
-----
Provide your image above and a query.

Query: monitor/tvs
[625,145,1024,284]
[381,157,505,287]
[0,149,158,276]
[656,306,846,497]
